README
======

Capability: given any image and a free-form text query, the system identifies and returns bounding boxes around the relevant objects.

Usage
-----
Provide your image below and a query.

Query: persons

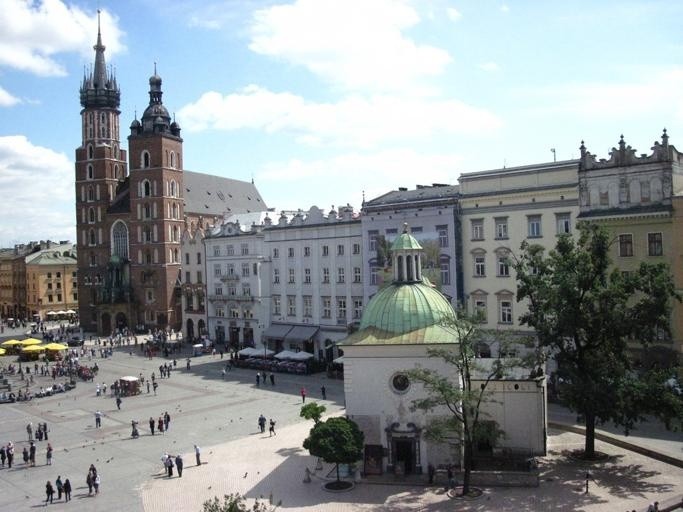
[302,388,306,403]
[257,414,274,437]
[0,316,191,403]
[1,422,53,468]
[161,454,183,477]
[256,371,274,385]
[428,463,434,483]
[191,335,243,360]
[95,410,102,429]
[131,412,170,438]
[321,385,326,400]
[87,464,101,497]
[448,470,453,488]
[45,476,72,502]
[194,445,201,466]
[116,397,122,410]
